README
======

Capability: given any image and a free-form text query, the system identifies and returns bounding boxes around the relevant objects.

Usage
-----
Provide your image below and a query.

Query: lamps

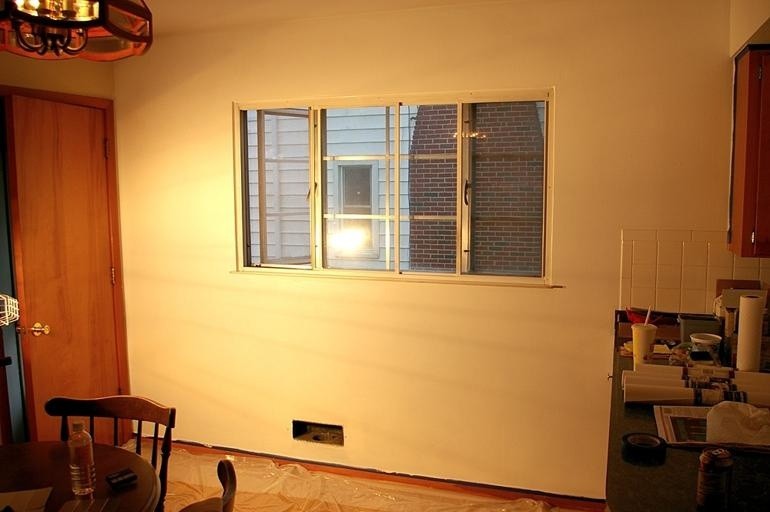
[0,1,154,64]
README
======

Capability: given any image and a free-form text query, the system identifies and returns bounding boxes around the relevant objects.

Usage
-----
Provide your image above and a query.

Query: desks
[0,438,162,511]
[603,308,770,511]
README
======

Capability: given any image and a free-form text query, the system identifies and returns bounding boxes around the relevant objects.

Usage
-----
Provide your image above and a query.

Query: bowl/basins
[626,306,661,325]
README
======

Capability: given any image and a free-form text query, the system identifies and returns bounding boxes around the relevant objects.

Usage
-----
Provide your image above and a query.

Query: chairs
[177,457,239,512]
[43,394,177,512]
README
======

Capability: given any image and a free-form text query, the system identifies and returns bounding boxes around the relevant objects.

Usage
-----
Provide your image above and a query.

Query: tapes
[621,432,667,467]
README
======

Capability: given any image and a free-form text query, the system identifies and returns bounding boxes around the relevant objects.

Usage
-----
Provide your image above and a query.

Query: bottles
[65,421,97,498]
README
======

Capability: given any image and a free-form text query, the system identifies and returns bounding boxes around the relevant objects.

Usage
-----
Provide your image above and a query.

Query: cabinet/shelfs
[729,48,770,258]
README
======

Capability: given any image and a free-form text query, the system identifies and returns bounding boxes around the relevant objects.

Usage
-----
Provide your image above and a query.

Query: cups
[689,332,722,357]
[630,322,657,370]
